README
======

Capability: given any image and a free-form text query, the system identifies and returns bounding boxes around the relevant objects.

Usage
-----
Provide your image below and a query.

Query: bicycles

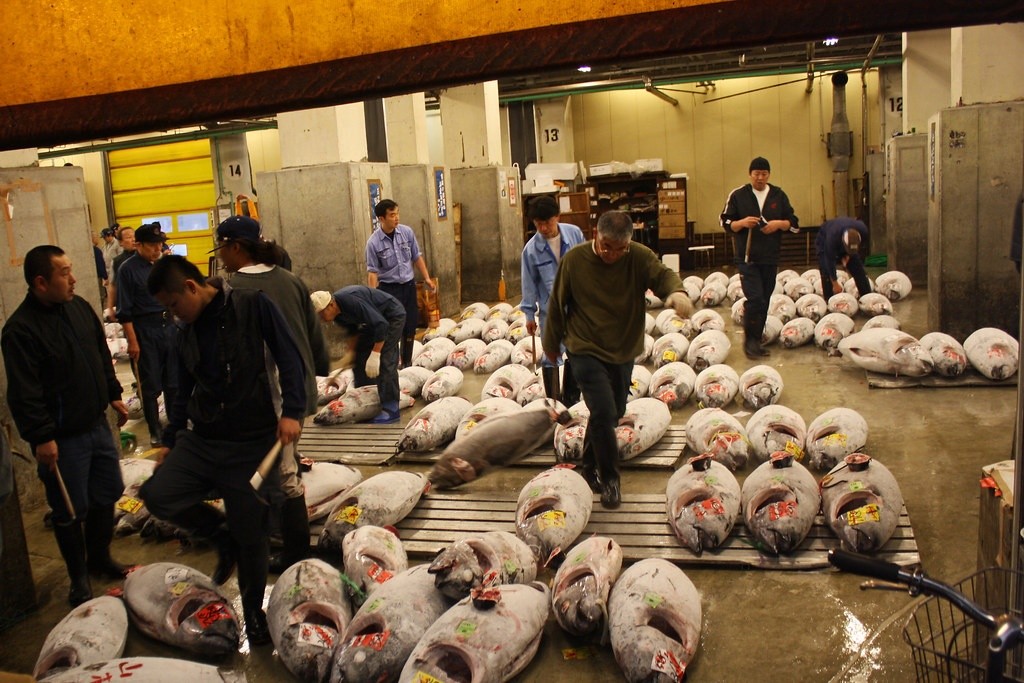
[828,548,1024,683]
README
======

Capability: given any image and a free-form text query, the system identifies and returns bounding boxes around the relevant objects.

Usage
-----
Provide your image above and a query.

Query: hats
[112,224,119,232]
[310,291,332,315]
[134,224,170,244]
[841,228,862,254]
[216,215,261,241]
[99,227,113,239]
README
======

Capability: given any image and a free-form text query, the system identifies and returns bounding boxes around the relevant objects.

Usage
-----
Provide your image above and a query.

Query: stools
[688,246,716,275]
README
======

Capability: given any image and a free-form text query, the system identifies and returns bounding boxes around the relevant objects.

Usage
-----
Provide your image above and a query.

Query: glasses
[597,238,630,256]
[534,217,558,229]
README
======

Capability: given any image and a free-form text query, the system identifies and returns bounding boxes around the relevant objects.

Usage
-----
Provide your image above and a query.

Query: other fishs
[314,303,786,492]
[110,457,432,558]
[665,403,903,556]
[647,266,913,355]
[32,525,702,683]
[103,306,130,361]
[834,327,1019,381]
[514,463,595,565]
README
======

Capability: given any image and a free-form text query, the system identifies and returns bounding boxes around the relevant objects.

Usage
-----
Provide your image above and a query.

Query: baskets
[901,567,1024,683]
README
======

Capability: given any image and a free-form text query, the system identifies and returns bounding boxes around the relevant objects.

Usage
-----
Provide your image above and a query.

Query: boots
[542,367,562,403]
[178,510,237,587]
[54,525,91,606]
[824,278,833,302]
[368,400,401,423]
[589,424,621,509]
[581,425,602,494]
[142,399,163,438]
[560,358,579,407]
[88,510,126,579]
[267,494,311,575]
[854,275,872,299]
[398,335,414,370]
[232,538,271,645]
[742,311,771,357]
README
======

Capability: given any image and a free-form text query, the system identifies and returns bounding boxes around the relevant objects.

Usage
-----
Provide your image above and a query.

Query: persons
[204,215,330,574]
[815,218,871,305]
[364,199,436,369]
[310,285,407,424]
[144,255,307,647]
[518,196,586,409]
[542,211,692,509]
[718,157,800,359]
[0,245,128,609]
[91,221,177,448]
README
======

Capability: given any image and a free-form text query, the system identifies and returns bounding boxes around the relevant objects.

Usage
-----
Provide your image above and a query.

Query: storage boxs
[661,254,680,277]
[658,215,686,227]
[970,459,1015,670]
[560,186,570,193]
[558,196,571,213]
[525,162,579,180]
[632,222,645,230]
[659,227,686,240]
[535,180,555,193]
[521,180,534,194]
[588,164,611,176]
[658,201,686,215]
[415,278,440,311]
[658,189,685,202]
[633,159,663,173]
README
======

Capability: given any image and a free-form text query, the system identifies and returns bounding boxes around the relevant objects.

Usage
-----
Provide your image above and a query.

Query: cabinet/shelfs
[586,171,690,272]
[523,192,592,242]
